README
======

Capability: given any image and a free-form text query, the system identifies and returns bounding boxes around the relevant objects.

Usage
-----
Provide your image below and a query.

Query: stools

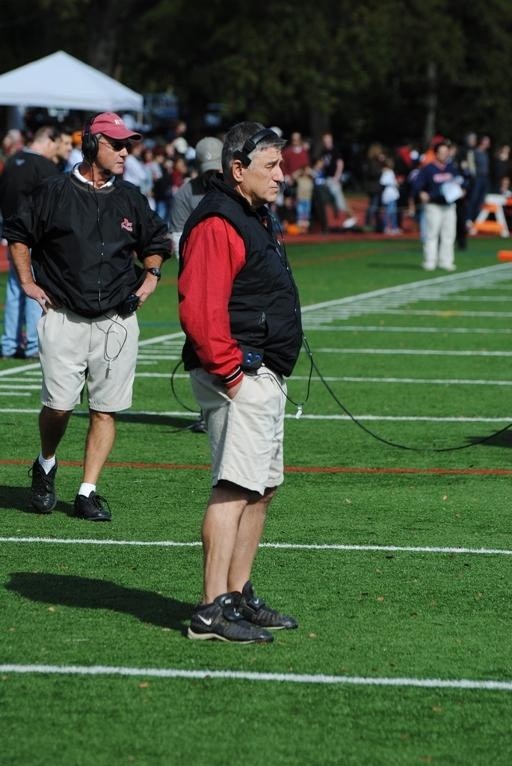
[465,194,511,237]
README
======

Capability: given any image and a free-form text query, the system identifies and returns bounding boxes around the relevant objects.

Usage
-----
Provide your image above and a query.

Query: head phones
[234,127,275,165]
[81,112,104,161]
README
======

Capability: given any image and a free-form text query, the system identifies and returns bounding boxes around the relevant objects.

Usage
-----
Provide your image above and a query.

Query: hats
[81,110,145,144]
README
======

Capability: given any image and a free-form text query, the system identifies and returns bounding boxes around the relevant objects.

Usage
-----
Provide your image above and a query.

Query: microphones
[92,169,110,175]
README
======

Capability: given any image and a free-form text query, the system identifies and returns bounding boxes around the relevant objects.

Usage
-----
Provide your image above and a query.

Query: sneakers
[227,581,299,631]
[28,454,60,515]
[186,593,275,646]
[73,491,114,523]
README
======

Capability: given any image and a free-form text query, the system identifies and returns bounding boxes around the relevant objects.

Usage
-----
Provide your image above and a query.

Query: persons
[0,124,87,360]
[177,119,304,649]
[120,135,196,220]
[2,111,171,521]
[167,138,223,433]
[274,118,512,272]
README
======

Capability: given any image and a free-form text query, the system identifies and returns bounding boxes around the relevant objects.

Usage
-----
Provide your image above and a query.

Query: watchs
[145,267,163,282]
